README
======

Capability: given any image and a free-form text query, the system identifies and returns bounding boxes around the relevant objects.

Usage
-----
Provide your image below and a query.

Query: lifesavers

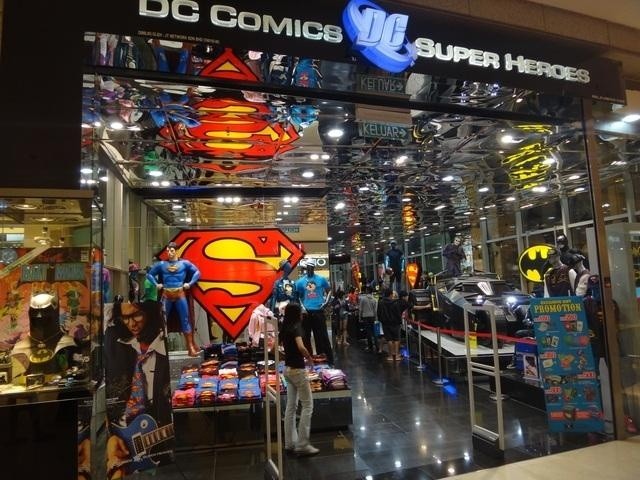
[316,259,326,266]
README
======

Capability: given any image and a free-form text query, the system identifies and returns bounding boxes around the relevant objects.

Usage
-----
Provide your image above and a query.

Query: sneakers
[284,444,320,458]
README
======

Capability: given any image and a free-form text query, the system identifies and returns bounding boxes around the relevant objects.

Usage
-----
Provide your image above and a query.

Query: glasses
[119,311,144,325]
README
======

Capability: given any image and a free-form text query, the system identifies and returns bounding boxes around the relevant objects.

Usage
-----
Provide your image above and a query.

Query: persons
[102,302,172,479]
[124,257,146,301]
[384,239,404,296]
[524,355,539,378]
[147,241,202,358]
[336,285,432,362]
[442,234,468,275]
[268,258,301,353]
[78,404,91,480]
[280,303,320,455]
[294,258,336,367]
[543,231,638,434]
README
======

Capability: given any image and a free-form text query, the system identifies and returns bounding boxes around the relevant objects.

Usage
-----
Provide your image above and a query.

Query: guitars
[107,413,174,479]
